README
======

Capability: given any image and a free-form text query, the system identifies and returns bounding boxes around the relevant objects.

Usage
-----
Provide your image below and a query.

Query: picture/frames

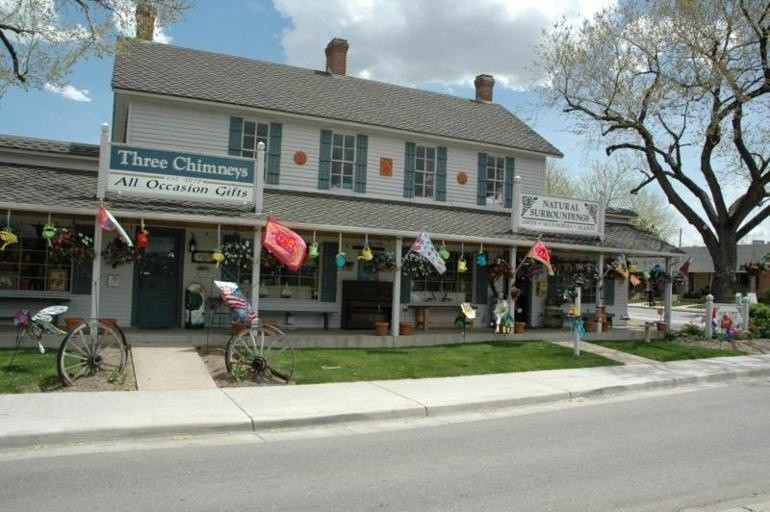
[46,268,70,292]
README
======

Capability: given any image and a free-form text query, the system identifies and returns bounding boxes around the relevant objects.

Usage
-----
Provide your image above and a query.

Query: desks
[407,303,480,332]
[1,292,71,330]
[257,298,340,329]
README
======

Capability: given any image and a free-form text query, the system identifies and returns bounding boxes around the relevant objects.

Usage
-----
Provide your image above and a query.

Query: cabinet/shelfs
[339,279,394,332]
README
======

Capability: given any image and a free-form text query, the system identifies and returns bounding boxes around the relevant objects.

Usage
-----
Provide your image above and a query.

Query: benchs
[564,312,616,327]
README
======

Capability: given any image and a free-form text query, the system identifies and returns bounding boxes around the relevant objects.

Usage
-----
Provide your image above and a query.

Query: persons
[645,288,656,307]
[492,292,509,333]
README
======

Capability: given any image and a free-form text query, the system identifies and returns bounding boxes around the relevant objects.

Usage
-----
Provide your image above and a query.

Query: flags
[567,298,587,339]
[97,204,134,248]
[213,280,264,331]
[609,253,652,287]
[709,306,719,334]
[411,226,446,276]
[678,258,692,279]
[526,237,556,276]
[262,216,308,272]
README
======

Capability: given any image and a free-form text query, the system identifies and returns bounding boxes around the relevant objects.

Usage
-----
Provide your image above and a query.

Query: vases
[657,323,668,332]
[583,321,597,333]
[98,318,118,337]
[399,321,413,337]
[231,321,251,339]
[261,320,278,336]
[375,321,389,336]
[65,317,84,334]
[514,321,526,334]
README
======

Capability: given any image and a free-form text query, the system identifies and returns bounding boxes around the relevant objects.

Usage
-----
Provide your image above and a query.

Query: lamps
[189,233,198,253]
